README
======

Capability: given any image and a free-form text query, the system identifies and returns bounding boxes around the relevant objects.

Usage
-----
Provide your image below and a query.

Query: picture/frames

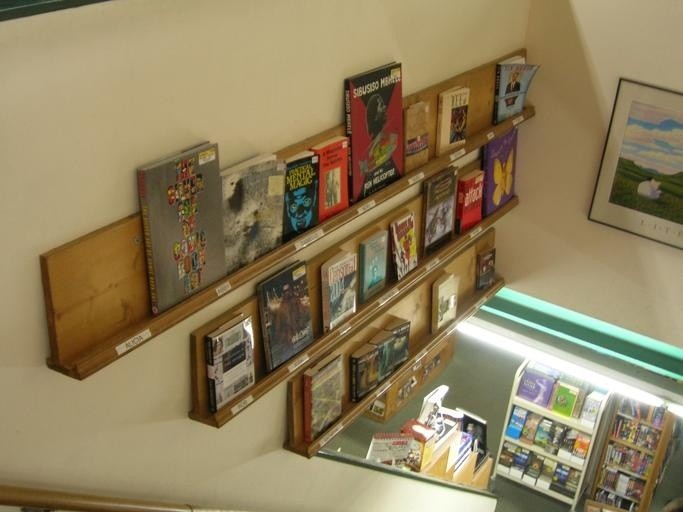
[585,76,683,251]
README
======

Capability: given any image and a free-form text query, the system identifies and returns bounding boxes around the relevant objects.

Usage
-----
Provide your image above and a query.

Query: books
[365,384,487,477]
[138,55,539,443]
[369,353,440,416]
[497,362,665,512]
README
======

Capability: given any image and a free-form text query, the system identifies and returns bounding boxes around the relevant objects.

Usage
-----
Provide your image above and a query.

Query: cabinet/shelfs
[492,354,674,512]
[40,47,538,458]
